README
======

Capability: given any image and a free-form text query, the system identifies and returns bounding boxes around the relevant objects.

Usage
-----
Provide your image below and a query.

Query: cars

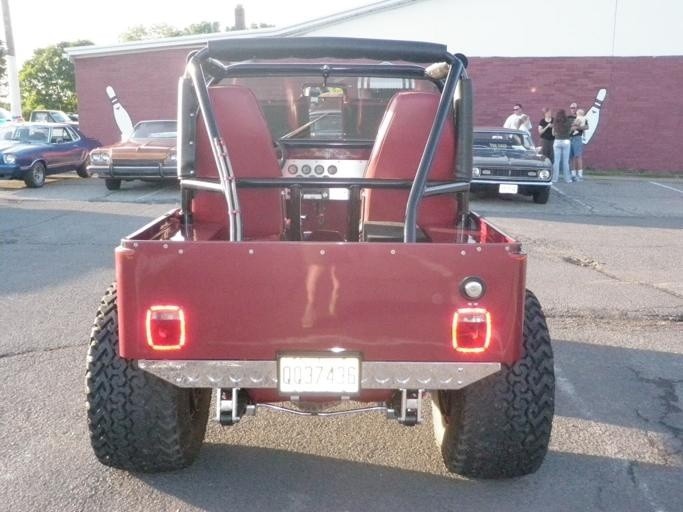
[471,126,554,203]
[0,108,179,189]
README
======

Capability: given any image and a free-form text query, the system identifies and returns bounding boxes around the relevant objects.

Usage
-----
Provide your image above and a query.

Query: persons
[503,101,590,183]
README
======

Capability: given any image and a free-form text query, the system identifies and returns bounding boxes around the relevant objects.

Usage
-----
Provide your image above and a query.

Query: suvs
[84,35,555,480]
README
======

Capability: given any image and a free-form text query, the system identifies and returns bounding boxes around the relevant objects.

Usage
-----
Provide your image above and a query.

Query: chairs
[191,85,285,242]
[361,91,457,242]
